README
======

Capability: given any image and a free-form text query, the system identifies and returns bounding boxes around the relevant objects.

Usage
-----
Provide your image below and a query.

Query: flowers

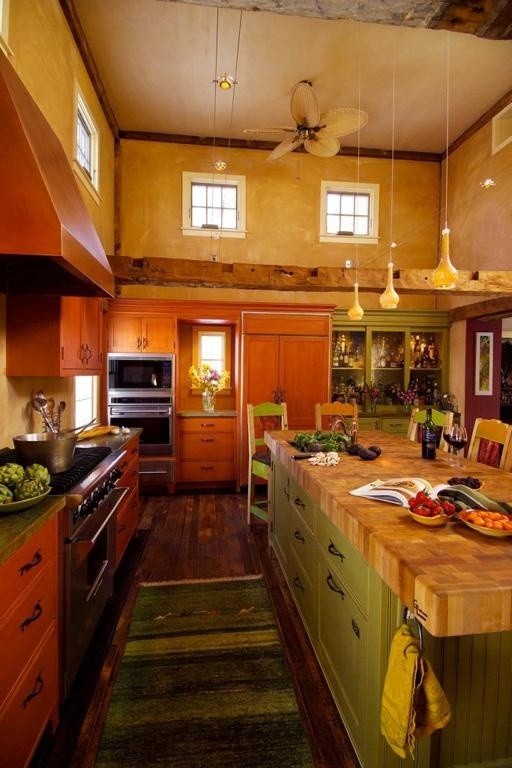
[187,360,231,395]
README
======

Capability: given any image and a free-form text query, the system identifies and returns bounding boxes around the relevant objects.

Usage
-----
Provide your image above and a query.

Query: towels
[378,619,455,763]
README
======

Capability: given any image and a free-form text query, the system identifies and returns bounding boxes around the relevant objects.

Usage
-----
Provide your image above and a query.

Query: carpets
[89,572,317,767]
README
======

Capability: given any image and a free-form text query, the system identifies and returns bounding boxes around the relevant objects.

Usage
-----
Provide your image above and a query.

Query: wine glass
[452,426,467,469]
[442,423,458,463]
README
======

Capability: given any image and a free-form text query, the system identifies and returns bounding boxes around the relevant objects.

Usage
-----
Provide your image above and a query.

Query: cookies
[468,512,512,530]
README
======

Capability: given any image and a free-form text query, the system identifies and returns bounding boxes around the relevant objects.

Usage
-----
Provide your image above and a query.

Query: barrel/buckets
[13,432,77,473]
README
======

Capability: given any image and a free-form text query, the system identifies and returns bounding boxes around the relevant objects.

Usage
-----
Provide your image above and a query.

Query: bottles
[421,408,436,458]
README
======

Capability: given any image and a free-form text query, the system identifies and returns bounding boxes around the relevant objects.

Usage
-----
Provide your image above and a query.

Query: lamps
[341,20,460,323]
[211,4,245,177]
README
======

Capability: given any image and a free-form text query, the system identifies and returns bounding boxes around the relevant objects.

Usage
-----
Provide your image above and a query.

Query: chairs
[312,398,362,438]
[244,399,290,529]
[405,401,455,455]
[464,415,511,474]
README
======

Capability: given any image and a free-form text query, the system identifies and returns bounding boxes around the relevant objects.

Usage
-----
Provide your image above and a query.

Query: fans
[239,78,371,163]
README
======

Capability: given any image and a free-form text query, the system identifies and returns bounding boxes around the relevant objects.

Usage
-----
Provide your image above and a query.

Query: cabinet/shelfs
[317,504,382,768]
[176,416,238,491]
[288,477,317,655]
[104,309,179,355]
[330,307,450,439]
[241,311,331,493]
[269,453,290,586]
[114,436,143,569]
[1,514,66,766]
[5,289,108,379]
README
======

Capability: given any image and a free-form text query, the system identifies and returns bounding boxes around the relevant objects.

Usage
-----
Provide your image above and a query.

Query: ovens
[110,406,173,493]
[63,488,127,697]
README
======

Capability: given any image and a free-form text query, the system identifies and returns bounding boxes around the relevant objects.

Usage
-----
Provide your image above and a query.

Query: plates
[456,508,511,536]
[408,506,453,527]
[1,485,52,514]
[446,474,485,492]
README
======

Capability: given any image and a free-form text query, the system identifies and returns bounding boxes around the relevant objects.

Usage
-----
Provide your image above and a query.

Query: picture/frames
[473,330,494,398]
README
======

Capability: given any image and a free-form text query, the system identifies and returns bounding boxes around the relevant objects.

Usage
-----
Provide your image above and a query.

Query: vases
[200,387,218,413]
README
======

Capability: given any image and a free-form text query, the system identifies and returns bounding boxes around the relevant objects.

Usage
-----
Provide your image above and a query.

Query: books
[348,476,453,509]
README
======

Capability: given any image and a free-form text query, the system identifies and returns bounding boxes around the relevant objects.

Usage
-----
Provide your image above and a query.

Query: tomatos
[409,489,456,516]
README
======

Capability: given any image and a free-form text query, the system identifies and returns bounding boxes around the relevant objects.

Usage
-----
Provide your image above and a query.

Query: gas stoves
[1,446,123,539]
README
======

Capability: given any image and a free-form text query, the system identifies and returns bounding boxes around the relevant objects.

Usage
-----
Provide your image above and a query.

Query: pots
[15,418,96,475]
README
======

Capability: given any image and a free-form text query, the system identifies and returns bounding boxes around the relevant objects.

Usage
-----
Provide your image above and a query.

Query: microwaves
[107,353,174,393]
